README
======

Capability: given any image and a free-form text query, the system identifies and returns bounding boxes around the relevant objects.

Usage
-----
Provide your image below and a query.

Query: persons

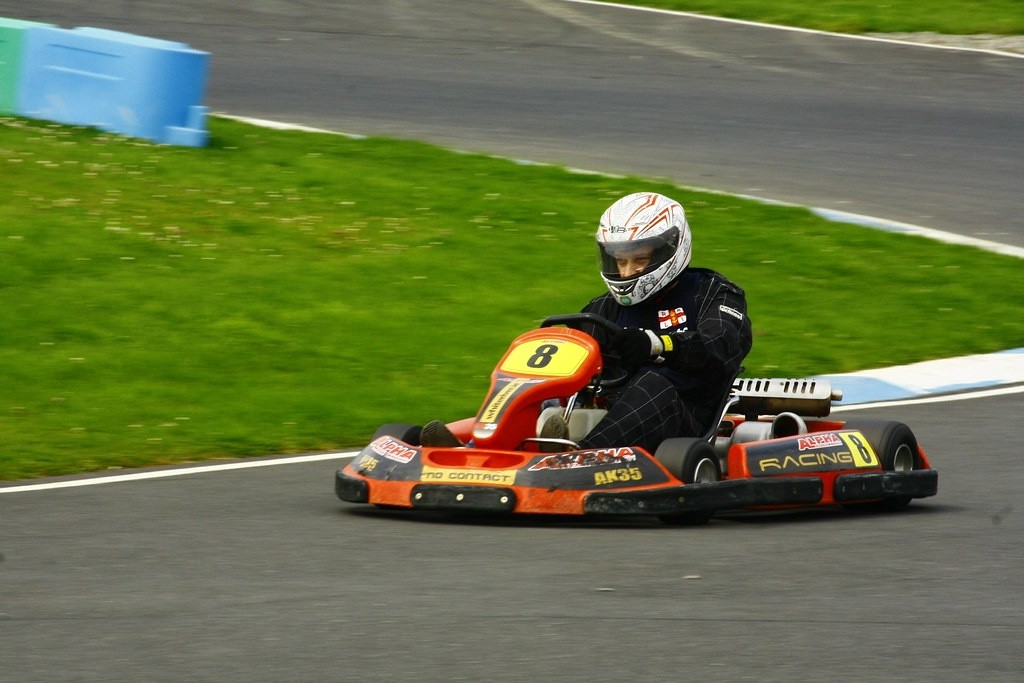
[420,191,752,453]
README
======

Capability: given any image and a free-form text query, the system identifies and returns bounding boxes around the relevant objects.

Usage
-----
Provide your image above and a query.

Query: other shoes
[538,414,570,453]
[420,419,466,447]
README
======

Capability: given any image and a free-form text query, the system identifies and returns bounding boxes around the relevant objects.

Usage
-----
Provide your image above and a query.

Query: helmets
[596,192,692,305]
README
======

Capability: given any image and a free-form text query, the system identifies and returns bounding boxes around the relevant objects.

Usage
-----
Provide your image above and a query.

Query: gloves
[611,326,664,364]
